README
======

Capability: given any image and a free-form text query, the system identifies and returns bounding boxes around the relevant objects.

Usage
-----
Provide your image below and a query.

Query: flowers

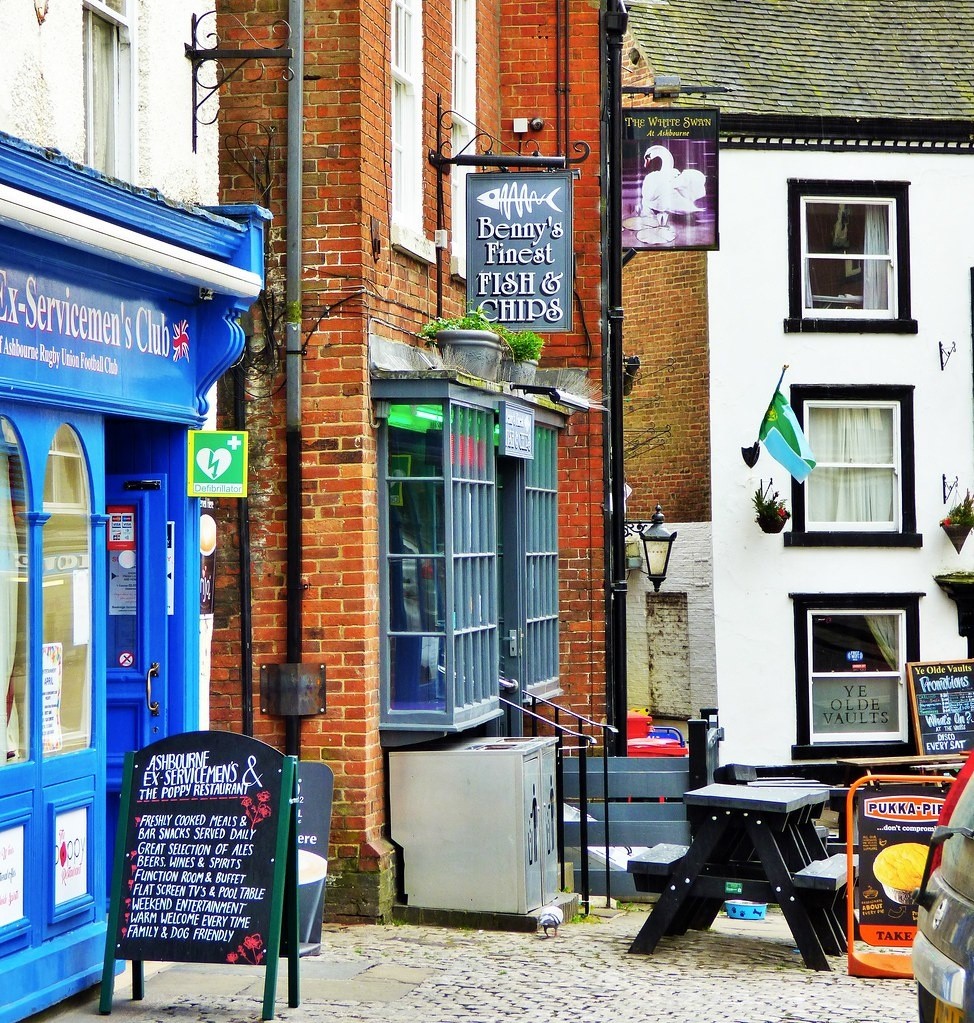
[753,478,791,521]
[938,488,974,529]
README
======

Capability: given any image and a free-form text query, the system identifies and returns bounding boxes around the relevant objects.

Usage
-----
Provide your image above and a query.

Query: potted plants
[499,324,544,384]
[418,298,511,381]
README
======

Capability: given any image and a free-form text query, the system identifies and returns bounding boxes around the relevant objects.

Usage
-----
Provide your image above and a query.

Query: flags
[758,388,816,484]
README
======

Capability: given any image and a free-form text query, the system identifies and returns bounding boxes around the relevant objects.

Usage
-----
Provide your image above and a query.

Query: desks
[627,781,849,972]
[837,750,971,790]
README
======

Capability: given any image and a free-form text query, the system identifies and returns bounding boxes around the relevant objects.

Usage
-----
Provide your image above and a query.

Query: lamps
[622,501,680,593]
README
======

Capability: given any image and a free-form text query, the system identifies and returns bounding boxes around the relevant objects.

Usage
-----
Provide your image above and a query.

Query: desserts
[873,843,932,905]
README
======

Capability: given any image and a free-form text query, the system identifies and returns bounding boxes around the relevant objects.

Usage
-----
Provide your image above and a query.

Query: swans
[642,145,707,214]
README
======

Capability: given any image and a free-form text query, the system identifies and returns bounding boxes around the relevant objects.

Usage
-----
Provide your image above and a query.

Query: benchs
[788,850,860,912]
[627,842,690,894]
